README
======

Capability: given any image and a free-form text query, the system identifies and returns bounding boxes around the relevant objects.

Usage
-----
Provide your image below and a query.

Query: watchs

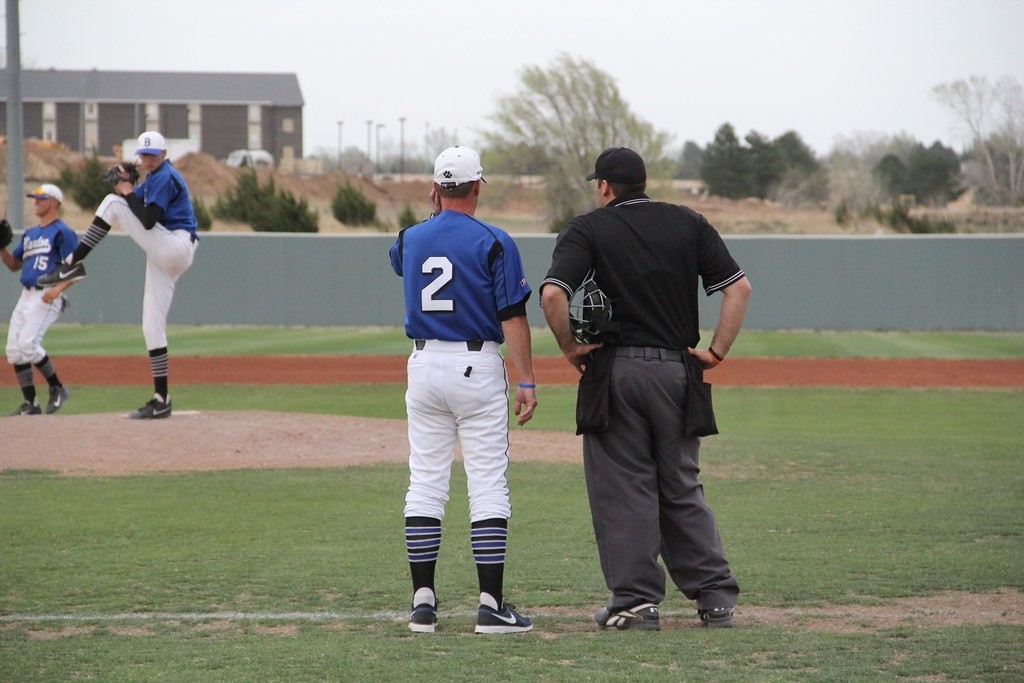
[429,211,439,219]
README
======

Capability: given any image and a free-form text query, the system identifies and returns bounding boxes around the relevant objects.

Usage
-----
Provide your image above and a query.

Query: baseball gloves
[0,220,14,250]
[106,162,139,185]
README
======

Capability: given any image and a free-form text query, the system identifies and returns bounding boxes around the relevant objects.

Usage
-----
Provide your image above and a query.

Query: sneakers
[475,602,533,634]
[11,402,42,417]
[36,259,87,286]
[46,385,68,414]
[408,598,438,632]
[700,607,733,627]
[128,393,171,420]
[594,603,660,630]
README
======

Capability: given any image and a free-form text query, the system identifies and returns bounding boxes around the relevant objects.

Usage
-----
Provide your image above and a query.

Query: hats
[586,147,646,184]
[433,145,486,188]
[26,184,62,203]
[135,131,165,155]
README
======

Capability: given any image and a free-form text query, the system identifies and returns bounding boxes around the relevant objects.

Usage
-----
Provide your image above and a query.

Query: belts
[415,339,483,351]
[190,234,195,243]
[614,347,683,361]
[25,286,43,290]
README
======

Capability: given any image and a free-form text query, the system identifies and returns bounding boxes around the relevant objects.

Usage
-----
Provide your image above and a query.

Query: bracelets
[708,347,722,363]
[55,287,61,292]
[519,384,536,388]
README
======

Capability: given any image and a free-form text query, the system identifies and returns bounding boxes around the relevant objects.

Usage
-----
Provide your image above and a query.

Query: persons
[389,146,537,634]
[0,182,78,417]
[36,130,200,419]
[538,148,752,630]
